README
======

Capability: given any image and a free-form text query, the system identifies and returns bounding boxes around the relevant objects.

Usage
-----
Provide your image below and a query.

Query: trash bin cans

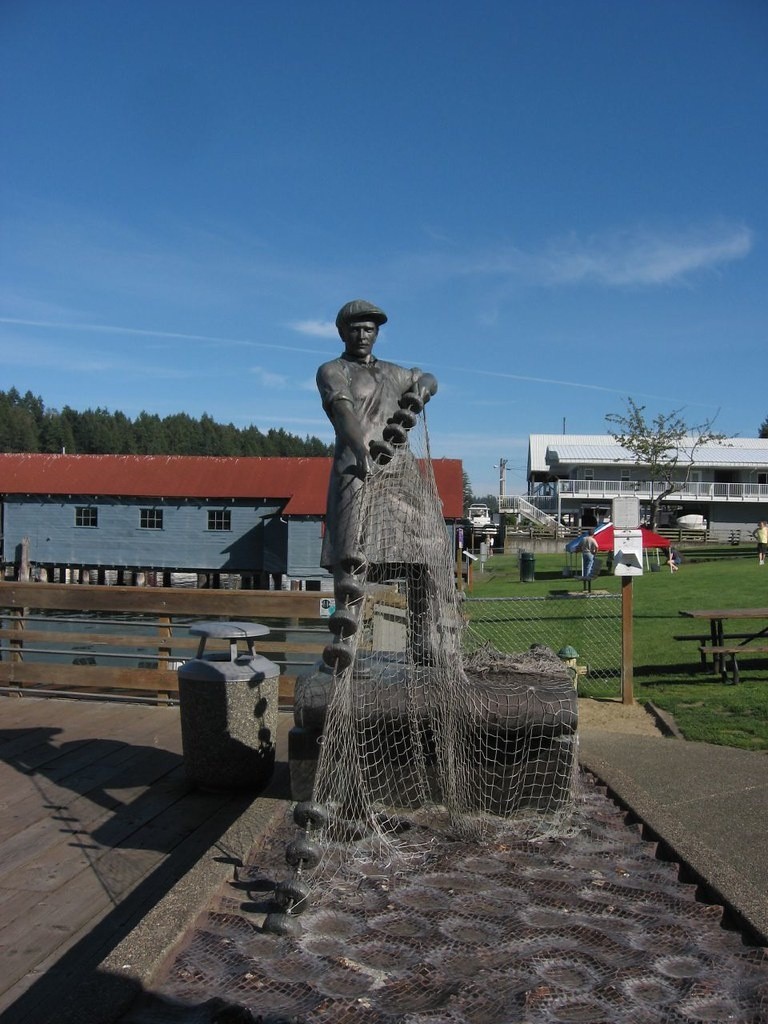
[519,553,536,582]
[177,620,281,791]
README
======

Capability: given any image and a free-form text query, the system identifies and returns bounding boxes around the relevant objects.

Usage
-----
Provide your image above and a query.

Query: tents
[565,522,671,575]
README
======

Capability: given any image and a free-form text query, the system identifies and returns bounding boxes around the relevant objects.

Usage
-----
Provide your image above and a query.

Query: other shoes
[759,561,764,565]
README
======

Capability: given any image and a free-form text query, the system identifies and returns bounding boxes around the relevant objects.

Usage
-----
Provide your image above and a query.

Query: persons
[582,530,599,576]
[316,299,453,679]
[485,535,494,556]
[753,521,768,566]
[606,550,614,576]
[666,545,681,571]
